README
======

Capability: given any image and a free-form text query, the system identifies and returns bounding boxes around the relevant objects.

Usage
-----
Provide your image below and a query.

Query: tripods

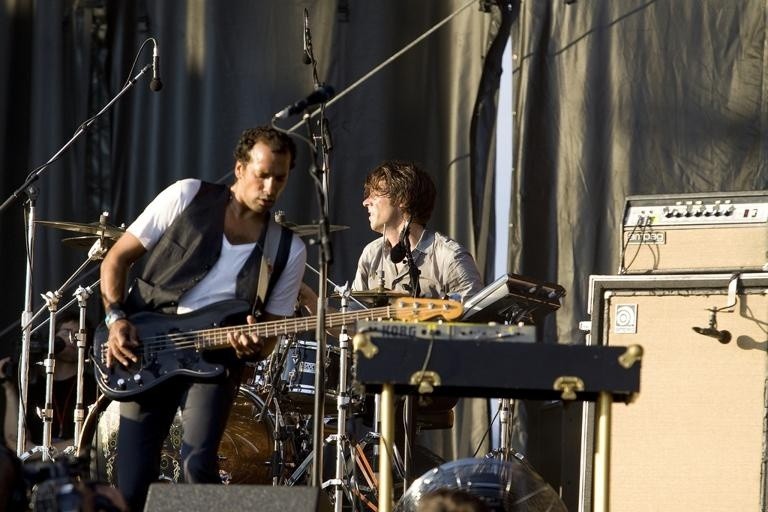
[471,409,547,499]
[16,323,61,465]
[287,351,369,512]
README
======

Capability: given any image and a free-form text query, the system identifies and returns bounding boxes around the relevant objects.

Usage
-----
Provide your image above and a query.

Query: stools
[411,407,454,434]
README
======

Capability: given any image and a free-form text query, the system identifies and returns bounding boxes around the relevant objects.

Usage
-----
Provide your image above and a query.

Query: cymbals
[32,219,124,280]
[274,220,350,237]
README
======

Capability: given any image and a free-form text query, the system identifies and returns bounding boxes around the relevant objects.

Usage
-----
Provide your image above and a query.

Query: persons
[99,124,309,511]
[0,310,97,460]
[343,157,486,311]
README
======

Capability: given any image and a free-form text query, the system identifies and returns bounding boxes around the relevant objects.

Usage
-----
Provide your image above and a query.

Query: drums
[254,361,280,393]
[78,376,312,510]
[277,340,365,415]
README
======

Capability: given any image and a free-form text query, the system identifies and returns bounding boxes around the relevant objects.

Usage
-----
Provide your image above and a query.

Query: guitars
[93,296,464,402]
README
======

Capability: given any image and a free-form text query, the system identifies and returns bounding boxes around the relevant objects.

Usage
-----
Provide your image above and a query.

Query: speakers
[142,483,334,512]
[579,272,768,512]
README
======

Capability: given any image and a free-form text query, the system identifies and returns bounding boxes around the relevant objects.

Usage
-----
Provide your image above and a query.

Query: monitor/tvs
[465,273,565,321]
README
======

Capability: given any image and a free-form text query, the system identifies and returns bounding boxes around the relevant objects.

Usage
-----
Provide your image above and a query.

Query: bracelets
[0,375,12,386]
[105,306,126,332]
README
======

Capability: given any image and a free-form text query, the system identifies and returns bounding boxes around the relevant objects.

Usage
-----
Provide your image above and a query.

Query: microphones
[389,221,410,266]
[276,83,337,120]
[689,320,735,349]
[148,43,164,95]
[299,15,315,68]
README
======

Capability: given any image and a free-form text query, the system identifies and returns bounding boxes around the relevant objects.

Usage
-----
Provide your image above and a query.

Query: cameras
[30,474,107,512]
